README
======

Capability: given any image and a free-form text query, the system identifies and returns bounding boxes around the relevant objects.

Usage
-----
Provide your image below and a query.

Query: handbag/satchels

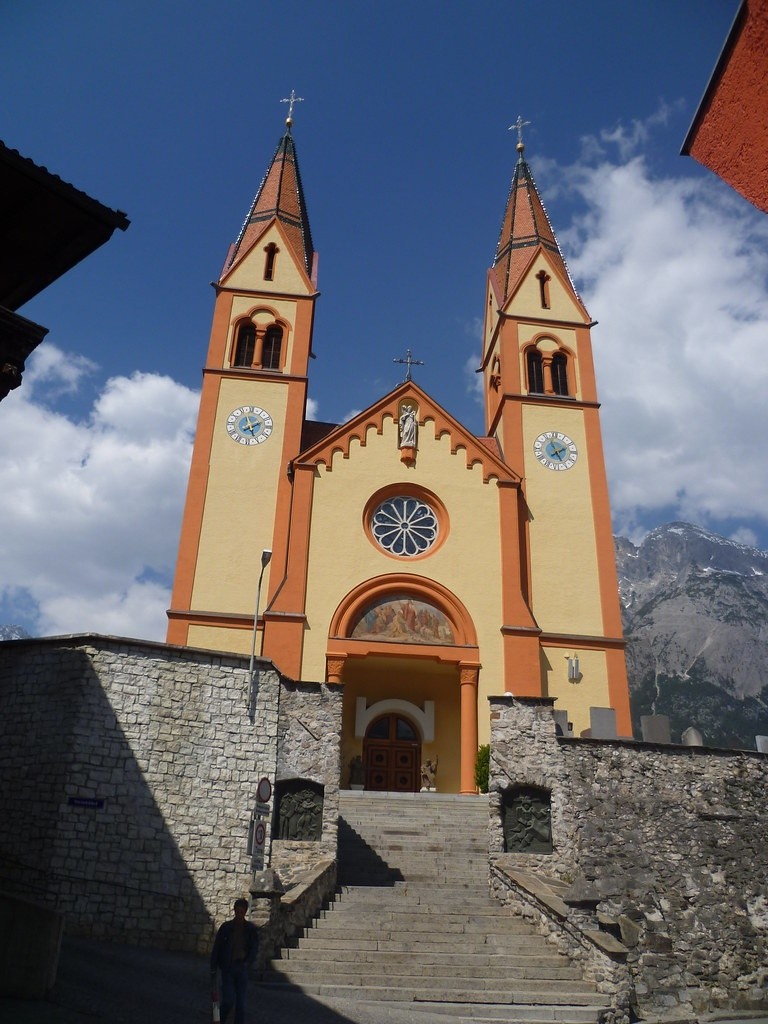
[211,974,220,1024]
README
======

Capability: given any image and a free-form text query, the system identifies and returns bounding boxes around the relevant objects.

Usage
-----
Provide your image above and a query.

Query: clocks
[226,406,273,445]
[534,431,578,470]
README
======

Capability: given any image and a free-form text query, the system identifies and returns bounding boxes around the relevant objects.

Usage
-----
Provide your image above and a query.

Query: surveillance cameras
[564,653,569,660]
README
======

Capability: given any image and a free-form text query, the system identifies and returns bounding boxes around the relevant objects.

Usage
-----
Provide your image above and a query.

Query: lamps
[568,659,579,679]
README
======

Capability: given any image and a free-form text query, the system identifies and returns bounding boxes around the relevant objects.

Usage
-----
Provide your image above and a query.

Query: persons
[508,797,548,853]
[350,755,366,785]
[291,793,321,841]
[211,898,259,1024]
[420,754,439,788]
[399,405,417,447]
